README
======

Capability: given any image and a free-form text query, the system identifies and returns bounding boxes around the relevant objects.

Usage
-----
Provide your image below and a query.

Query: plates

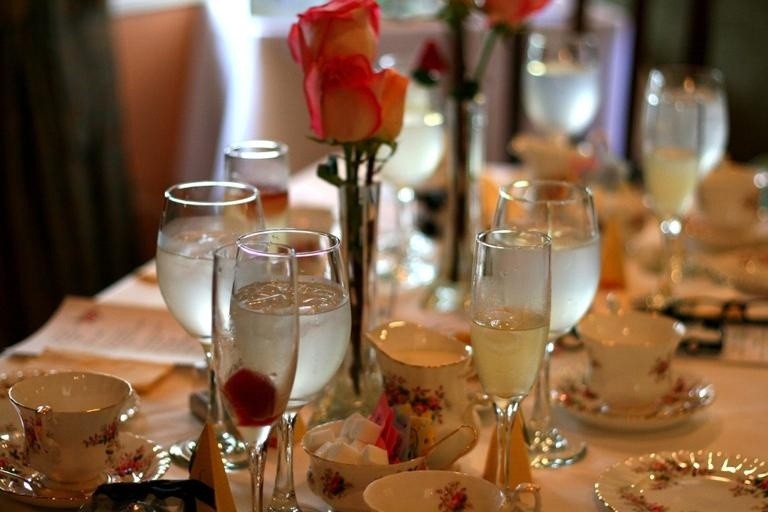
[2,371,139,435]
[595,449,768,512]
[553,368,716,430]
[3,431,170,508]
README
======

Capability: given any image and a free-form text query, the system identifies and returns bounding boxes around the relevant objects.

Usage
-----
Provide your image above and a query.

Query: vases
[306,179,387,421]
[423,93,497,316]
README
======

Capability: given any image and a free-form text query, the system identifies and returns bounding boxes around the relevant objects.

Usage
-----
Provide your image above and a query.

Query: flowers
[416,0,566,283]
[288,0,411,396]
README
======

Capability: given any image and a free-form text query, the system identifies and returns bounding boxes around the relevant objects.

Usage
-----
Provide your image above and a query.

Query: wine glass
[156,182,265,469]
[374,84,450,292]
[235,230,352,512]
[492,182,602,464]
[470,229,551,512]
[629,61,731,310]
[211,243,297,512]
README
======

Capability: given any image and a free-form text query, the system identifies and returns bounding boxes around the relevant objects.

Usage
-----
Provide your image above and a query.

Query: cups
[361,320,474,460]
[577,314,687,410]
[224,141,291,227]
[304,417,476,510]
[520,30,603,142]
[363,469,544,512]
[9,370,134,481]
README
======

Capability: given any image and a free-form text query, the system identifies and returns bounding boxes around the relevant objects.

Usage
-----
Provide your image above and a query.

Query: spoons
[1,469,85,499]
